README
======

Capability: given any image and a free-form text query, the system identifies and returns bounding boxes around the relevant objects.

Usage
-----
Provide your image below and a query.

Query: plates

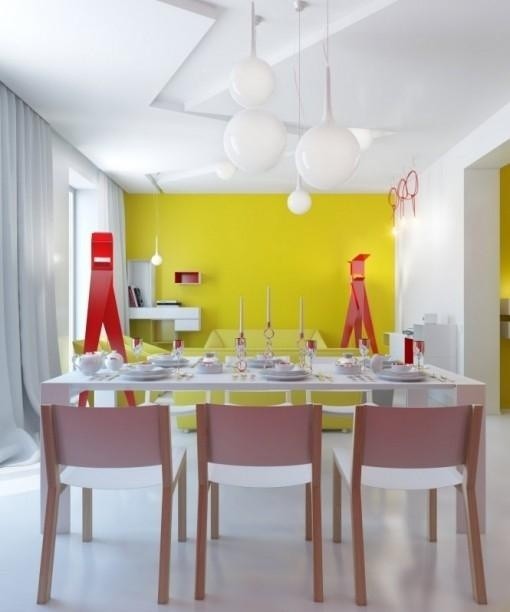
[262,369,306,381]
[122,368,174,381]
[380,370,425,381]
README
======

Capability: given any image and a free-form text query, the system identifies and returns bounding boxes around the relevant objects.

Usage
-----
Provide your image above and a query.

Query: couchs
[204,328,325,349]
[108,331,171,397]
[172,347,369,431]
[72,337,157,408]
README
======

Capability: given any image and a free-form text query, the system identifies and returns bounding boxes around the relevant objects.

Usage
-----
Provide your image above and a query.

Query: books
[155,300,182,308]
[128,285,144,308]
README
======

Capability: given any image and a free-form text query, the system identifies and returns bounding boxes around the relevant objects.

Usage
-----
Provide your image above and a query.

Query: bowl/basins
[391,364,412,373]
[135,365,154,371]
[274,362,295,371]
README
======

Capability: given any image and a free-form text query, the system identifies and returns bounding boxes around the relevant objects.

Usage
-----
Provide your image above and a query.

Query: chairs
[332,405,487,607]
[36,404,187,604]
[304,355,380,415]
[196,403,322,601]
[222,354,293,406]
[133,354,211,416]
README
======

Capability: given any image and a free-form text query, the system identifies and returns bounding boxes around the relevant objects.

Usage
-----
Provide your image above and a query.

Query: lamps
[226,0,274,109]
[286,1,312,215]
[223,15,286,173]
[294,1,361,192]
[150,172,162,267]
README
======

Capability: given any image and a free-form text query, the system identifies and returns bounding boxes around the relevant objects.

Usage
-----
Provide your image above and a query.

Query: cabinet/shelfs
[125,261,201,350]
[389,324,458,374]
